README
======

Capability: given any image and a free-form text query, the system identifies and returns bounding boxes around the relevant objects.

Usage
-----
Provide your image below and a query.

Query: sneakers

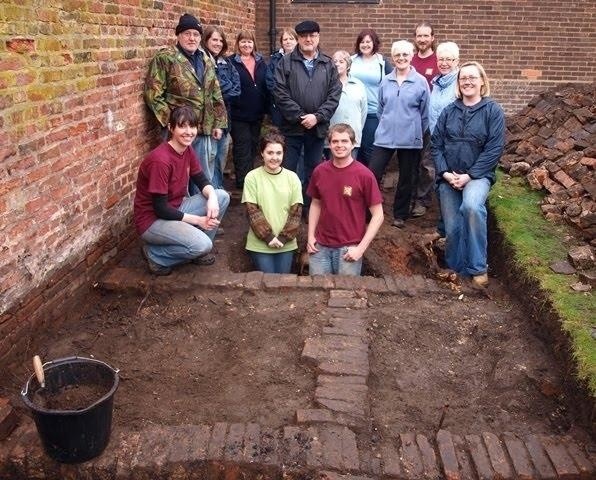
[216,225,225,235]
[142,245,172,275]
[393,218,405,228]
[411,200,426,217]
[473,273,488,290]
[193,254,215,265]
[424,193,434,208]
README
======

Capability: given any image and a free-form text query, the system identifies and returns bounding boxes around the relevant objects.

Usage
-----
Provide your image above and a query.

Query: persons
[135,106,231,276]
[432,61,505,287]
[143,13,461,234]
[306,123,385,275]
[241,128,304,273]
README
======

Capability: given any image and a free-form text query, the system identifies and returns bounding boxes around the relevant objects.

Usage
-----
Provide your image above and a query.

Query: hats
[175,14,203,35]
[295,21,320,34]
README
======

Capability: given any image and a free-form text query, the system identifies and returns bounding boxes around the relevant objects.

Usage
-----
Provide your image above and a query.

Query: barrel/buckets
[20,355,121,465]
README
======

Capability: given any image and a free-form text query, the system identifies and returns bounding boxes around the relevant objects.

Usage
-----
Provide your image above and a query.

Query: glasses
[436,58,457,63]
[458,76,482,81]
[393,53,409,59]
